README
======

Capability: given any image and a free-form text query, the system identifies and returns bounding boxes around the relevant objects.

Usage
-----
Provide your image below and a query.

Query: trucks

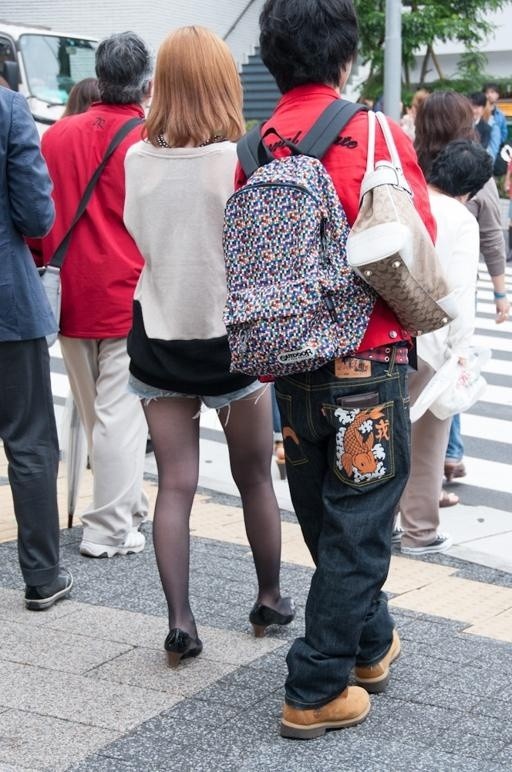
[0,17,146,155]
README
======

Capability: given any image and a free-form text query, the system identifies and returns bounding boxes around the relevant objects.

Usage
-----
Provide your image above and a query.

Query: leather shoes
[354,629,401,694]
[440,490,459,507]
[279,685,371,739]
[24,567,73,610]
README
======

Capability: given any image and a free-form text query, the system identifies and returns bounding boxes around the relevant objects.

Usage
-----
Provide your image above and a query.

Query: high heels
[444,462,466,484]
[163,628,202,666]
[249,597,296,637]
[274,443,288,482]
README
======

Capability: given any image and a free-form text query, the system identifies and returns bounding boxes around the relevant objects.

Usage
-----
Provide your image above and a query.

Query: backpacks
[222,99,375,378]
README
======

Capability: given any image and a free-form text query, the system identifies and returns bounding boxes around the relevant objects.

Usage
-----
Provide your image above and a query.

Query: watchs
[494,289,507,299]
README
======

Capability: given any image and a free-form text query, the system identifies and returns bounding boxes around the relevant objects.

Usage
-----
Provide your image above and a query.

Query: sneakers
[400,536,452,555]
[79,529,147,559]
[390,529,404,544]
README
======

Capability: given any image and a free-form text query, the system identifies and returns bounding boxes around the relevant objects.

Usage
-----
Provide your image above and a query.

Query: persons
[387,137,496,557]
[0,80,76,614]
[408,90,512,484]
[119,24,300,671]
[234,0,458,742]
[30,27,186,563]
[56,77,99,123]
[362,80,512,266]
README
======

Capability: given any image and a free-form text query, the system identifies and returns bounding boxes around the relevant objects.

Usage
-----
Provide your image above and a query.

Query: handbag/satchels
[36,265,62,347]
[344,111,459,340]
